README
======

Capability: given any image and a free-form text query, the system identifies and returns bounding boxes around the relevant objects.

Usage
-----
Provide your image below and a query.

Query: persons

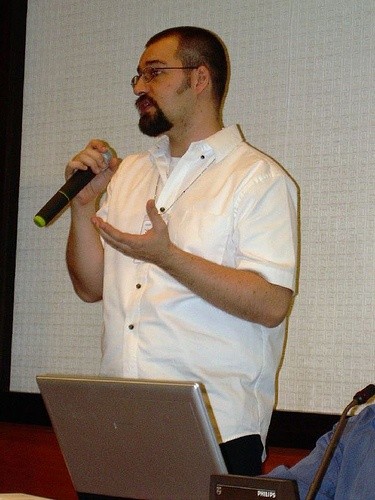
[65,27,300,478]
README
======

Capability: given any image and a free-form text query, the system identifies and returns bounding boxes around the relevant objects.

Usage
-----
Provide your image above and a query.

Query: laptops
[33,374,231,499]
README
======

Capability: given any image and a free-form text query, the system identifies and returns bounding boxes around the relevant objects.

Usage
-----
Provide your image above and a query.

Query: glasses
[131,66,198,85]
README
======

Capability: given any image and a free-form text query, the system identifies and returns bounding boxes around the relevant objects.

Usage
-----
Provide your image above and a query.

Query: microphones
[33,148,117,228]
[303,383,375,500]
